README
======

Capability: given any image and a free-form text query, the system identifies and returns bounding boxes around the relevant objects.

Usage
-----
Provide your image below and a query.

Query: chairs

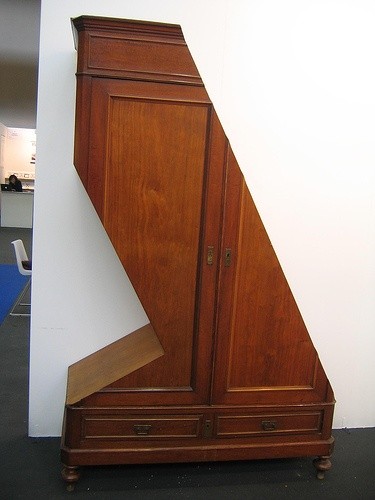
[9,240,30,317]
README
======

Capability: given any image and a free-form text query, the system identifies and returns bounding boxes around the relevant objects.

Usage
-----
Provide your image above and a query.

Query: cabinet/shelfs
[59,15,336,493]
[5,177,34,190]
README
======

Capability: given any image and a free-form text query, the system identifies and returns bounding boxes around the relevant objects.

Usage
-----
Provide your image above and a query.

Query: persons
[8,175,22,192]
